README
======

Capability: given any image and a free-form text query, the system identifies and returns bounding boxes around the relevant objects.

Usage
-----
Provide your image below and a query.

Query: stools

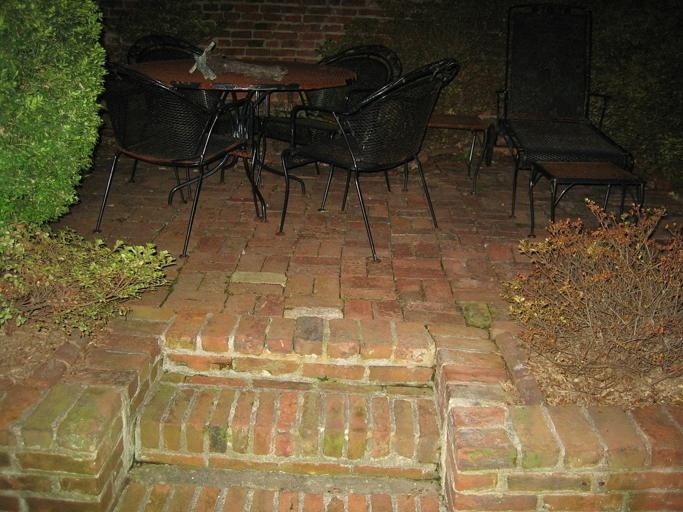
[526,160,648,239]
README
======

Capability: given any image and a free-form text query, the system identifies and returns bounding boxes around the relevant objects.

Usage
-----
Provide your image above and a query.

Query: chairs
[298,44,405,193]
[95,57,262,260]
[279,56,470,262]
[126,34,242,204]
[482,4,638,219]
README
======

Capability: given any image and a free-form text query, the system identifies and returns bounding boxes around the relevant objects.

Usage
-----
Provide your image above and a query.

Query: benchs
[427,112,488,176]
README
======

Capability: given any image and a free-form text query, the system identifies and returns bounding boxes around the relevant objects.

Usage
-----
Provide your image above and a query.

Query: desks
[125,60,359,223]
